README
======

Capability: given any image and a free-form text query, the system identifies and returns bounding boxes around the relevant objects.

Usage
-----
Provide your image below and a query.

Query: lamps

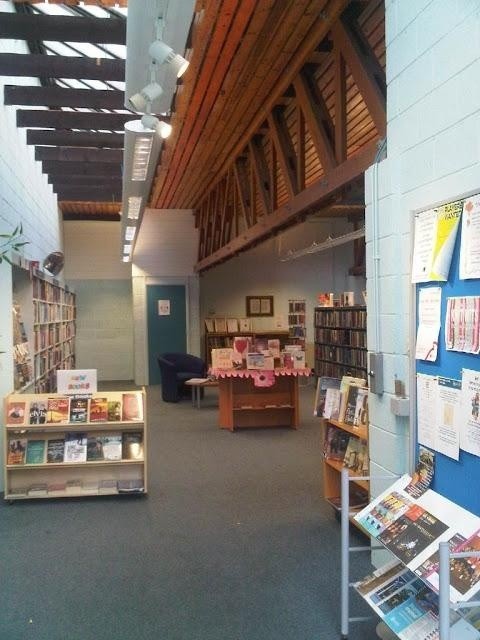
[157,352,205,403]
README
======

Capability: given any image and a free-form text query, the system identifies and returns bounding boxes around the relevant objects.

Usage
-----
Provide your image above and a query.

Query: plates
[314,307,367,387]
[340,467,480,640]
[3,387,147,504]
[0,251,77,393]
[205,330,289,376]
[315,418,371,539]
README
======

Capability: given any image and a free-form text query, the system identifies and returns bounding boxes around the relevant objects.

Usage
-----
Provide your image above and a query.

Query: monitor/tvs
[340,474,480,640]
[11,275,76,392]
[312,289,369,476]
[326,489,368,511]
[205,300,308,370]
[7,392,145,501]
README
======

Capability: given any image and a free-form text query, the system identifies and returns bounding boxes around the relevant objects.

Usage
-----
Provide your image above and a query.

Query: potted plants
[246,296,274,317]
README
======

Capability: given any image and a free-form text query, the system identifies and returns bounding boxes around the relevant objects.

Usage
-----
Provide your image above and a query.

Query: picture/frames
[208,369,311,432]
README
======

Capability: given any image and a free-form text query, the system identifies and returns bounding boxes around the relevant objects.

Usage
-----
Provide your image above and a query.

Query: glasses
[128,2,192,141]
